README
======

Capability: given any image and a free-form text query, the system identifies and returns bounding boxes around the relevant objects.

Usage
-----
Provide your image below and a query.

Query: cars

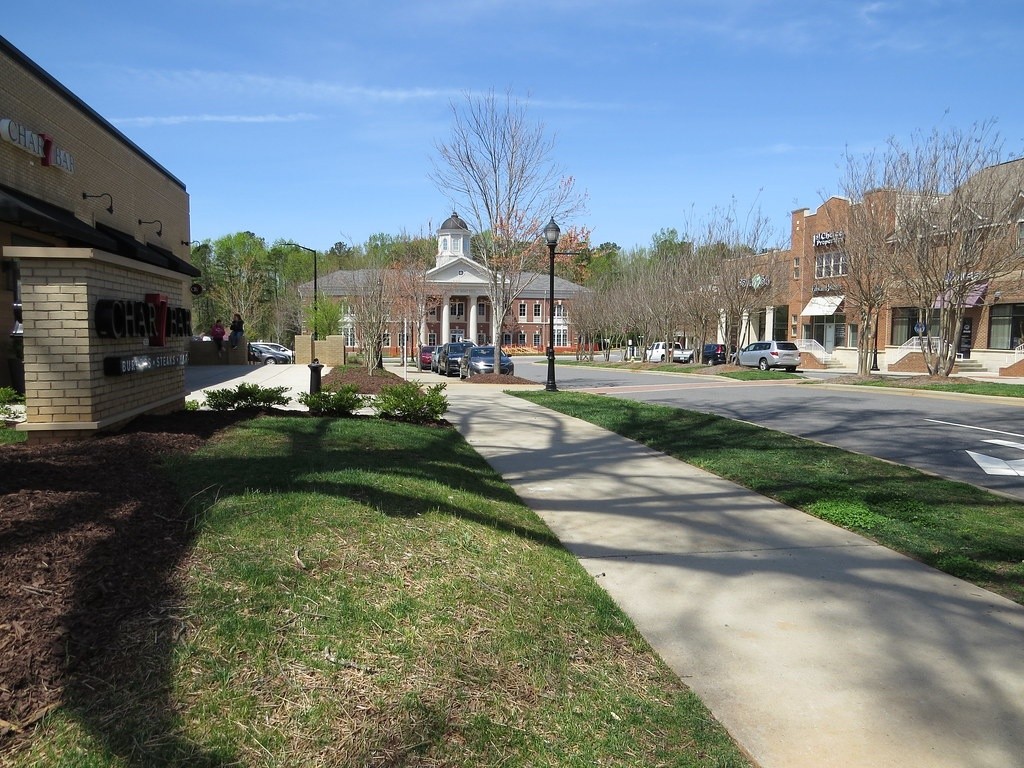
[459,345,515,380]
[247,342,295,365]
[431,344,444,373]
[416,345,435,370]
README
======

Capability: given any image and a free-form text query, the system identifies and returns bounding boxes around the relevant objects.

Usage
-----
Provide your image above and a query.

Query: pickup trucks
[643,341,694,364]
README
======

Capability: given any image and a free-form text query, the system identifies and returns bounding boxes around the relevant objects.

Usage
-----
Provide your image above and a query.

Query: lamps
[138,219,162,238]
[181,240,200,251]
[82,192,113,214]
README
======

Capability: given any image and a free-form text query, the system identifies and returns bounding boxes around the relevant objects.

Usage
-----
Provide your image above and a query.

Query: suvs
[438,340,482,377]
[731,340,802,373]
[687,344,736,367]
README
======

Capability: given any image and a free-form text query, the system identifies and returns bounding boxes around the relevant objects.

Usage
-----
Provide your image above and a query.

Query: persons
[212,320,226,357]
[230,314,244,351]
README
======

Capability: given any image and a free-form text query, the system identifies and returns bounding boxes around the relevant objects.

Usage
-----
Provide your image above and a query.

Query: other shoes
[232,346,238,352]
[218,353,221,357]
[221,347,225,351]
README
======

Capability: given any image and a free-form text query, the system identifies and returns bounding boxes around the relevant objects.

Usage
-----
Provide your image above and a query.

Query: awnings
[932,284,989,308]
[800,295,845,316]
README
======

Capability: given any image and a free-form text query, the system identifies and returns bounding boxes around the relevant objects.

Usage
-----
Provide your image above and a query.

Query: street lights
[279,243,318,341]
[543,216,560,392]
